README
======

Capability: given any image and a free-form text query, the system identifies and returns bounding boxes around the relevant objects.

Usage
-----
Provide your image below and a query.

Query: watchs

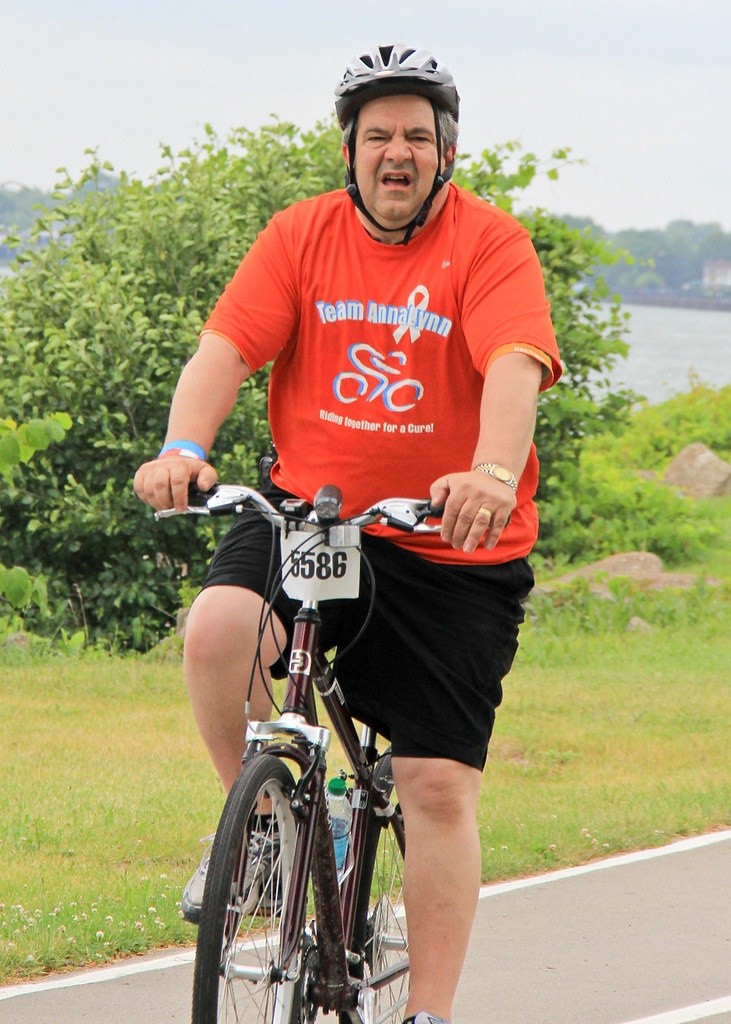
[473,463,518,495]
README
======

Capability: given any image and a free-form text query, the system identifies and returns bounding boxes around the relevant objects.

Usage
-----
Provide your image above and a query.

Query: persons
[133,45,563,1024]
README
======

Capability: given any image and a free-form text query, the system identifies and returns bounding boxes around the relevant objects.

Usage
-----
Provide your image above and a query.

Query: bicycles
[146,482,511,1024]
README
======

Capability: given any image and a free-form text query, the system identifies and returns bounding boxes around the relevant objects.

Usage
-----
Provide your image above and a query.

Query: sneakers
[186,831,290,923]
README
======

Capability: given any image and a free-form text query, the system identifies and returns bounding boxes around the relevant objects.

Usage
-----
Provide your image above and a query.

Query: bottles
[327,776,355,880]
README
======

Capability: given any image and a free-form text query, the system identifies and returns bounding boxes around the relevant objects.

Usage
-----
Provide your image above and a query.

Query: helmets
[334,43,461,120]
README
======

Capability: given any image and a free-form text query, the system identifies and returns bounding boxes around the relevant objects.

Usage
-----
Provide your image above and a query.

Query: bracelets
[156,440,206,462]
